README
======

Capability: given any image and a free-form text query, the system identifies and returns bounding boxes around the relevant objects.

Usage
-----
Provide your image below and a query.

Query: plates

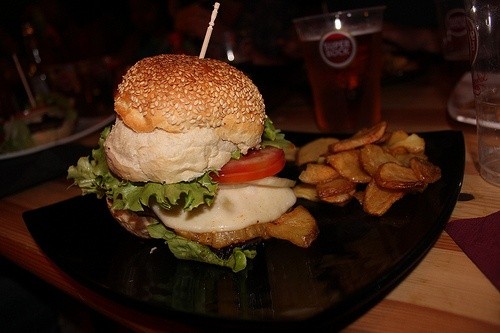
[447,71,500,129]
[22,131,465,333]
[0,115,115,160]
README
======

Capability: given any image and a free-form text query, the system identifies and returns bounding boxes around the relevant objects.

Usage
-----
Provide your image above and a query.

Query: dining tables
[0,66,500,333]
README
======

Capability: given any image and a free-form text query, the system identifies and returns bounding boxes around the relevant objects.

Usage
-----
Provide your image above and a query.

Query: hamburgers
[2,89,77,151]
[65,54,296,273]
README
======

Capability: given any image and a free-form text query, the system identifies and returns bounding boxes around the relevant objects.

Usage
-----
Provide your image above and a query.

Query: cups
[464,0,500,188]
[293,6,384,134]
